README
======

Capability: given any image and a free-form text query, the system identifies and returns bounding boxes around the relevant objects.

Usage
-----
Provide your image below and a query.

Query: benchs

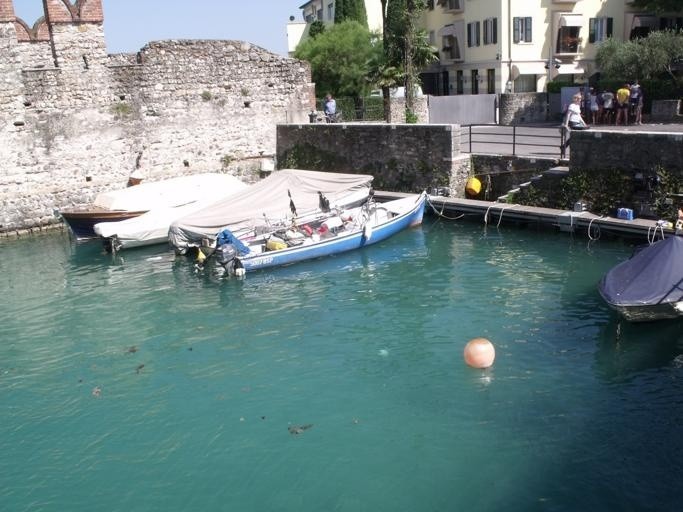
[647,97,682,124]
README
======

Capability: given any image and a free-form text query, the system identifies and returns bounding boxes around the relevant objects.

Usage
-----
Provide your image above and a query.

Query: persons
[558,92,589,155]
[677,207,683,228]
[573,78,644,126]
[324,92,336,123]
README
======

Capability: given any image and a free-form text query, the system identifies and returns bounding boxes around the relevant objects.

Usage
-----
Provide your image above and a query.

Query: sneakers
[560,145,565,155]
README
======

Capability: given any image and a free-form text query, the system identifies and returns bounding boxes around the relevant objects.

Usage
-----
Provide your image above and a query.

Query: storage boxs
[617,207,633,220]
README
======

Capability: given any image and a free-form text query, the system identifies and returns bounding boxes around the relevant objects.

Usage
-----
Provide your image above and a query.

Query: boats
[592,221,683,332]
[591,315,683,384]
